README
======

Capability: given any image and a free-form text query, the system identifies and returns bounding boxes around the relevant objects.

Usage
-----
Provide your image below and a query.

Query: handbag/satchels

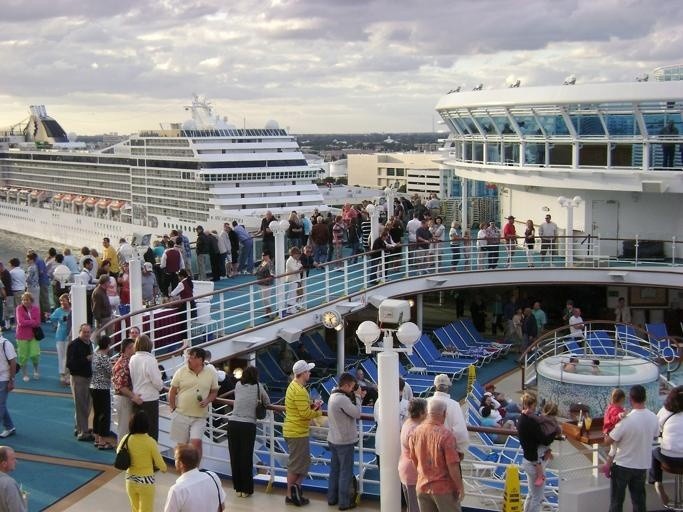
[114,447,131,470]
[257,404,266,420]
[298,287,304,295]
[481,247,488,251]
[33,326,45,340]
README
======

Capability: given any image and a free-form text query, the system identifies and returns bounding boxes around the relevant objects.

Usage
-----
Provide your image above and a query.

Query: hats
[144,262,153,272]
[176,268,186,275]
[508,216,516,219]
[293,360,315,374]
[434,374,452,385]
[486,384,496,389]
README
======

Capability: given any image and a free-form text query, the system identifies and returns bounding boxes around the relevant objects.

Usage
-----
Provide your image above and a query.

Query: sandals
[75,428,114,450]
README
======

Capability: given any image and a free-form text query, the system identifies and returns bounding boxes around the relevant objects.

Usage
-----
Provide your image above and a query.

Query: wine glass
[575,415,593,438]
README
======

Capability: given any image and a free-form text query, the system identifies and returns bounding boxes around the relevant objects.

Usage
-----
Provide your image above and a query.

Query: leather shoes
[234,489,249,497]
[329,502,357,510]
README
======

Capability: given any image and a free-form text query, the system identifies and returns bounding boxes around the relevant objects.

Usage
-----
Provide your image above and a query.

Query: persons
[0,190,72,512]
[64,324,469,512]
[477,219,502,269]
[452,285,683,512]
[523,220,535,267]
[503,215,518,268]
[538,214,557,267]
[73,192,470,342]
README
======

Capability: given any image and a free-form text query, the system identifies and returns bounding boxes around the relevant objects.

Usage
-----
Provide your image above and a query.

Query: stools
[191,320,218,341]
[661,463,683,511]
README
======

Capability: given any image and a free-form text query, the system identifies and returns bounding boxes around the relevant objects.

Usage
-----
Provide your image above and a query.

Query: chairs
[458,380,560,511]
[524,322,683,390]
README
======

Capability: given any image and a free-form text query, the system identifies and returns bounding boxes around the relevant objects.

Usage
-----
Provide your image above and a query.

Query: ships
[0,94,329,250]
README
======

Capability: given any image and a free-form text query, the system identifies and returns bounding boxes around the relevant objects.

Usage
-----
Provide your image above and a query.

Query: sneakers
[0,428,16,438]
[23,374,40,381]
[2,327,11,332]
[210,275,228,281]
[286,485,310,506]
[601,464,611,479]
[655,483,669,504]
[535,475,545,486]
[286,306,307,314]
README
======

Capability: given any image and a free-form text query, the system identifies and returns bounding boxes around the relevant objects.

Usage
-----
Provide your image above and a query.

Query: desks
[113,307,181,349]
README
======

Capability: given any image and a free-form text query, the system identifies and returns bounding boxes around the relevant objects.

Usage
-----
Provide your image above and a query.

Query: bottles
[144,294,160,308]
[196,388,202,404]
